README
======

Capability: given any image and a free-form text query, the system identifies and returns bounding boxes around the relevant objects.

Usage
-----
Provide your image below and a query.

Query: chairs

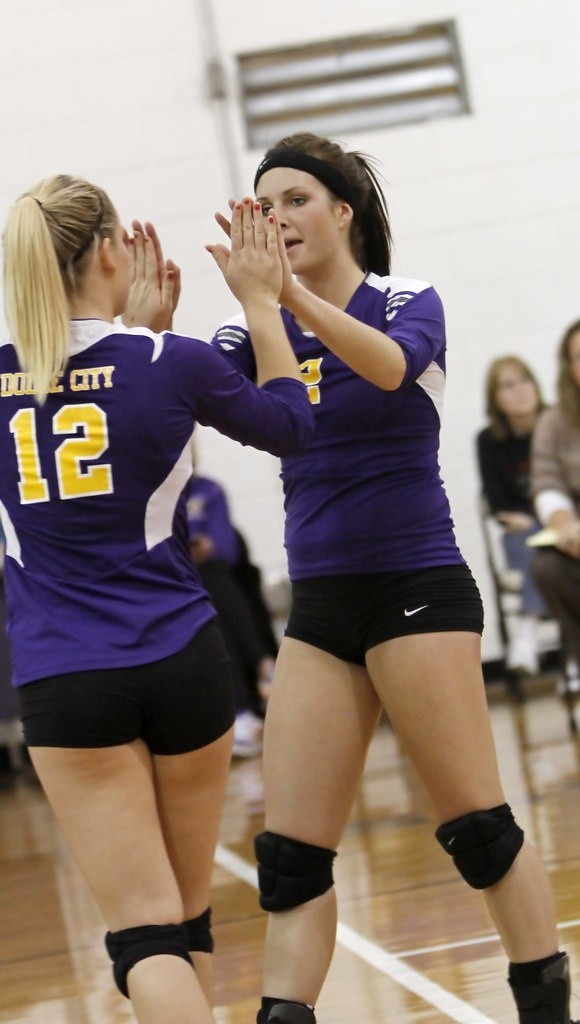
[478,495,580,797]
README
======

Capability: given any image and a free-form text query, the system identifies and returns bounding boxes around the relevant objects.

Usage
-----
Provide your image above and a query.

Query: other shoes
[504,619,540,674]
[230,710,266,756]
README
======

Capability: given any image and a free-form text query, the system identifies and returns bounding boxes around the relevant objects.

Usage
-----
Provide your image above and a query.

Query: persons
[2,133,577,1024]
